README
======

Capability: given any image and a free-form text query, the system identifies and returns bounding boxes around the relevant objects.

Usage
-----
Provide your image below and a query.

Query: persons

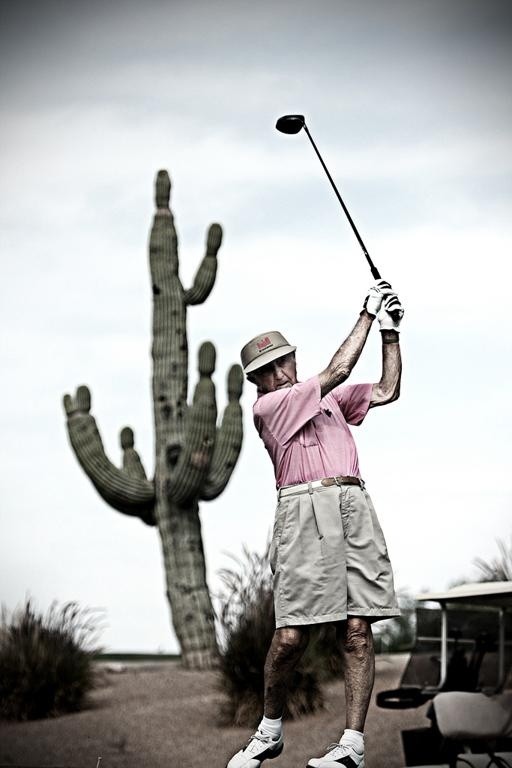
[224,279,404,768]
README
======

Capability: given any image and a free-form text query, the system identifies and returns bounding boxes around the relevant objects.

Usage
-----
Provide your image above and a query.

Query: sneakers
[226,731,284,768]
[308,743,365,767]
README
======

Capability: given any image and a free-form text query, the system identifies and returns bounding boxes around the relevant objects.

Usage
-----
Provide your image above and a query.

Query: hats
[240,331,296,375]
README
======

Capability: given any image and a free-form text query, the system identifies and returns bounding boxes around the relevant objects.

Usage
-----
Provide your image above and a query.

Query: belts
[280,476,365,497]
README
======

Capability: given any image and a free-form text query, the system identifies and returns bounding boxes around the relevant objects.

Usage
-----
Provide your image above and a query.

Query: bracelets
[382,336,400,345]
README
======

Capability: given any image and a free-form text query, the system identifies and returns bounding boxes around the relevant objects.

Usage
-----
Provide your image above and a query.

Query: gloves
[360,279,405,333]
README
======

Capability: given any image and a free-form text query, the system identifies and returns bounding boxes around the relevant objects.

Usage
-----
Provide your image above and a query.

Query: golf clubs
[277,116,403,322]
